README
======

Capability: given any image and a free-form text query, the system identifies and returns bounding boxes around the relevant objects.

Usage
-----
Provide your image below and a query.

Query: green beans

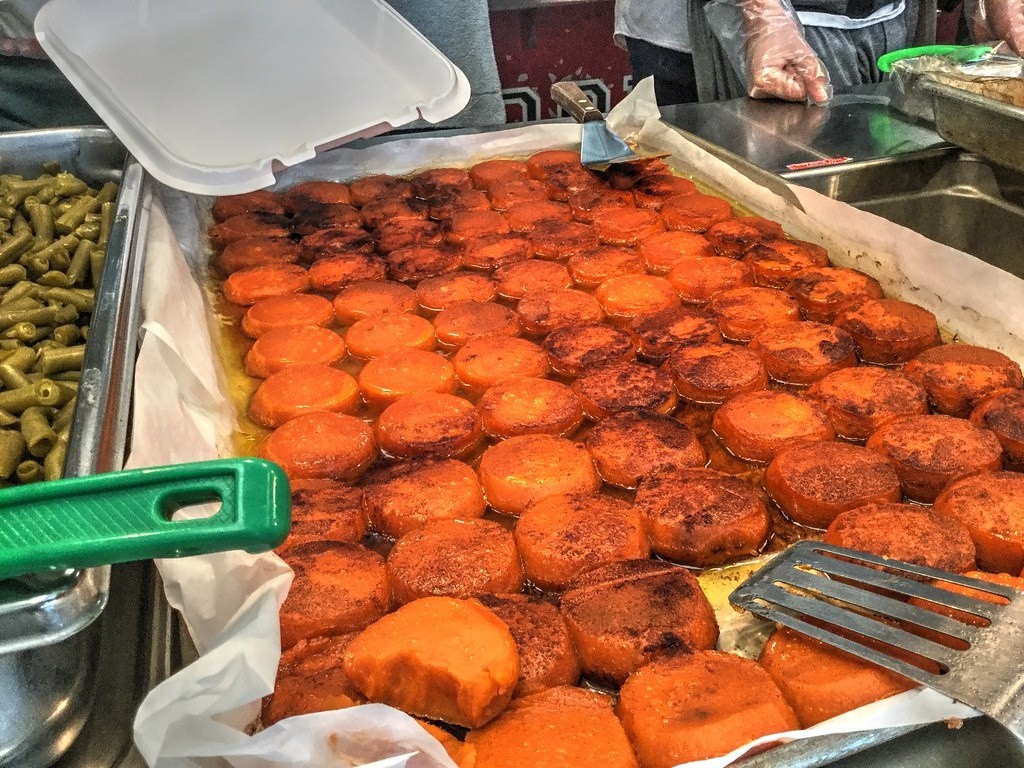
[0,159,122,488]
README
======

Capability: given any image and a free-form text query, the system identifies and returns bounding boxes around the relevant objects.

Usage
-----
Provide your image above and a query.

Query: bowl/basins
[876,44,992,73]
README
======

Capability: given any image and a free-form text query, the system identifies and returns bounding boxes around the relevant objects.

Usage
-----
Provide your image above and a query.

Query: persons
[610,0,1024,104]
[0,0,508,141]
